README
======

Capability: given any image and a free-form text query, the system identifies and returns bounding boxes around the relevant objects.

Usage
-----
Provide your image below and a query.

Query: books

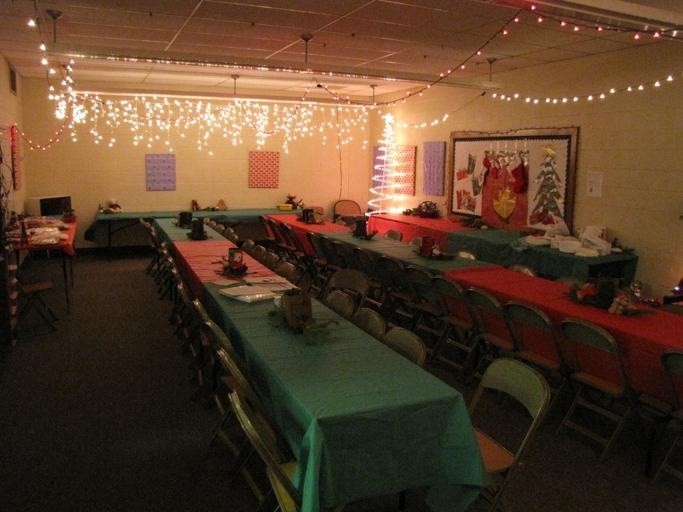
[211,272,297,305]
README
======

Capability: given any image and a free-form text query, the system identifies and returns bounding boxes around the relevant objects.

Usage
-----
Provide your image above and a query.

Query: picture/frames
[448,126,579,235]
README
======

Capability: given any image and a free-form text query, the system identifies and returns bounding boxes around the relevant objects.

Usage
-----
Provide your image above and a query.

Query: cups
[189,218,204,236]
[227,247,244,270]
[178,211,194,228]
[420,236,434,256]
[355,220,367,235]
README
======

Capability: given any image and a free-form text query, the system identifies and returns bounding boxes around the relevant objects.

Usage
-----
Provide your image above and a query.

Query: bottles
[97,201,105,215]
[19,220,30,248]
[298,207,323,225]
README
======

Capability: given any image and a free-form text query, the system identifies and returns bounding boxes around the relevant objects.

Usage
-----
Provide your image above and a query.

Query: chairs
[17,280,61,334]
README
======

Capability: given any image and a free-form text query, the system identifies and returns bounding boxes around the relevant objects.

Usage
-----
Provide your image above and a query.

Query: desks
[0,212,78,314]
[84,208,302,264]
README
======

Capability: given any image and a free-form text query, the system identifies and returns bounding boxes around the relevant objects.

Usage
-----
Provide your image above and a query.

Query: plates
[187,232,208,240]
[176,223,193,229]
[224,266,249,276]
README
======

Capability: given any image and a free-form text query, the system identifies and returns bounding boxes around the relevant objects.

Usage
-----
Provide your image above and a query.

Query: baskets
[278,286,313,321]
